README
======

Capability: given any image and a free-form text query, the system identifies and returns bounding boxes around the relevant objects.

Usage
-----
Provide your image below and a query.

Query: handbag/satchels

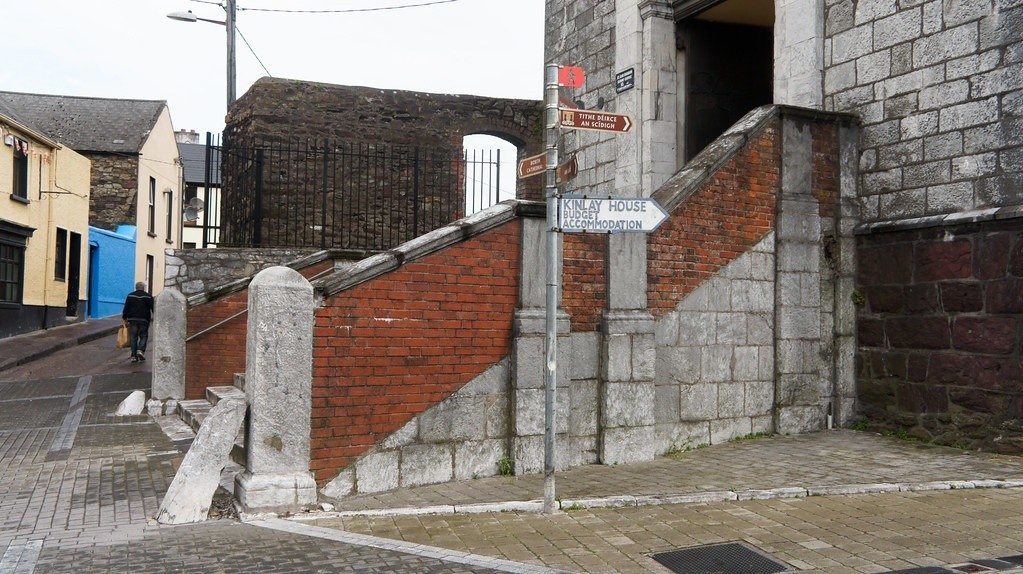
[116,321,132,348]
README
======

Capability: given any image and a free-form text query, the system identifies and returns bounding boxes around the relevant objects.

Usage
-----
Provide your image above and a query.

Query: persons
[122,282,153,362]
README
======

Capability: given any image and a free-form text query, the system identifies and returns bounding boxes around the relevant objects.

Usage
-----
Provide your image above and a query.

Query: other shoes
[131,356,137,362]
[136,350,146,361]
